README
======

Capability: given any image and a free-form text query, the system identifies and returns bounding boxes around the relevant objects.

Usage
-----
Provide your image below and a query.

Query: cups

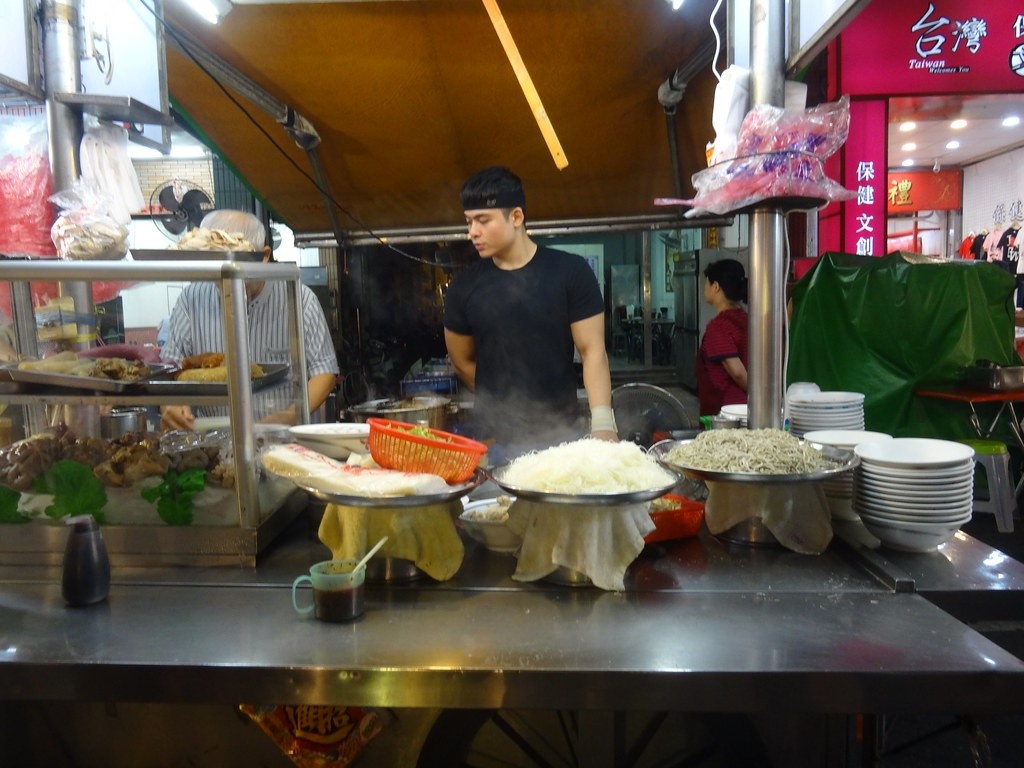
[293,558,368,623]
[711,415,742,432]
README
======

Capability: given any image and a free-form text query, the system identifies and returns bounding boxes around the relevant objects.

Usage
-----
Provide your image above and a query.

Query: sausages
[17,343,179,377]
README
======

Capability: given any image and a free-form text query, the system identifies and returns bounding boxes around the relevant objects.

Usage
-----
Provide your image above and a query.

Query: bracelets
[590,406,618,433]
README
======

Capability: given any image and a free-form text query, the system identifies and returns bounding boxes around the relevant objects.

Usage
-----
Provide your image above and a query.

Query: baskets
[643,493,704,542]
[367,417,486,483]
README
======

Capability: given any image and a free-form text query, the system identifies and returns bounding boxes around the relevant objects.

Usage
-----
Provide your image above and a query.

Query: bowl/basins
[967,366,1024,394]
[786,381,976,553]
[459,498,520,553]
[721,404,748,428]
[289,423,372,458]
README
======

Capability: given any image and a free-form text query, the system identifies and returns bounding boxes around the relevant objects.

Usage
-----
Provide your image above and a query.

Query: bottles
[60,513,111,606]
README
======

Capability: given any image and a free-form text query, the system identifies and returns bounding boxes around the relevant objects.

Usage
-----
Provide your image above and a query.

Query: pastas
[663,428,822,474]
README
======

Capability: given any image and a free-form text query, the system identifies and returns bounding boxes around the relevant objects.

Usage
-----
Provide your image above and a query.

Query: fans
[148,177,217,244]
[608,382,692,455]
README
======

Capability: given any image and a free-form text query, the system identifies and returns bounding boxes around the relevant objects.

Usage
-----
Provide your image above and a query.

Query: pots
[347,394,451,434]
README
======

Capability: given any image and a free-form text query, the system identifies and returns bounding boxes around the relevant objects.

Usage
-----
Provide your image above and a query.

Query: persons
[694,259,748,430]
[444,167,619,466]
[158,209,339,432]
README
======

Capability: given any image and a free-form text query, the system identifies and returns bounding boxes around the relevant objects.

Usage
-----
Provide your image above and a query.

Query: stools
[955,436,1018,533]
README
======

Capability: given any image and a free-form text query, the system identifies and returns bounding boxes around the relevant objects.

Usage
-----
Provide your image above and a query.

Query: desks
[913,384,1024,503]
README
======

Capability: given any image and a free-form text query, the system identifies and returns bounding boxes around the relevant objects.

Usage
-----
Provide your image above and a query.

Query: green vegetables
[374,423,478,483]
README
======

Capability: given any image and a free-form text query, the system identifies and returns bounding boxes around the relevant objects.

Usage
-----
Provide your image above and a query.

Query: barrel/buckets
[102,407,148,439]
